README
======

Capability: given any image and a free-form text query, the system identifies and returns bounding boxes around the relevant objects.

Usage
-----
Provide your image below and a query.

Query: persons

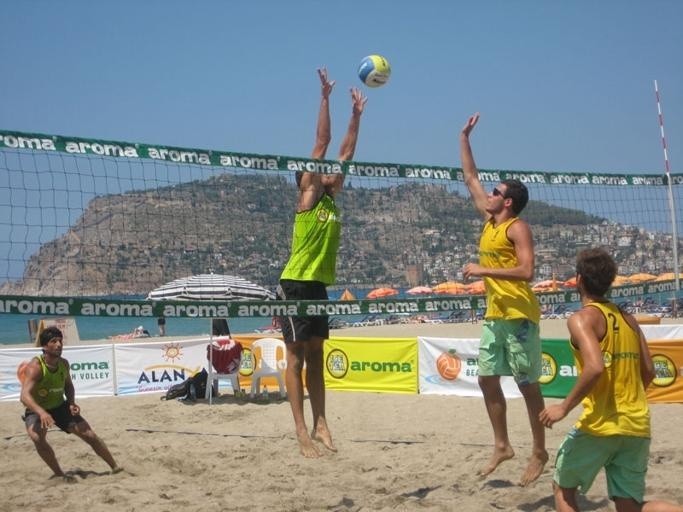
[538,247,683,512]
[158,318,166,337]
[460,112,549,486]
[134,326,143,335]
[20,326,119,476]
[275,67,367,459]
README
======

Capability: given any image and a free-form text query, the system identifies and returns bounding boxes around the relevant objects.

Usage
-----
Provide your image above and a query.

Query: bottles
[262,385,268,401]
[241,389,245,398]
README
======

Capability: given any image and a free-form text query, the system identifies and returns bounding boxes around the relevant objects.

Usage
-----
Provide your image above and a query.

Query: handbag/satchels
[165,368,214,398]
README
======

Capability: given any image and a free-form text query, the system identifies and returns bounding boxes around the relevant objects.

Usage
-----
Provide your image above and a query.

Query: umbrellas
[341,289,355,301]
[146,274,278,406]
[435,271,683,296]
[406,286,432,296]
[367,288,398,298]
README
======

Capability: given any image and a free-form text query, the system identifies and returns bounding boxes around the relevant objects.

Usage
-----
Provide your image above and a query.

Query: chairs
[204,338,287,398]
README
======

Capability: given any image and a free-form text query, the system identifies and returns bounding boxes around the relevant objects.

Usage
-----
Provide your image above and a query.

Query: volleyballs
[357,54,390,89]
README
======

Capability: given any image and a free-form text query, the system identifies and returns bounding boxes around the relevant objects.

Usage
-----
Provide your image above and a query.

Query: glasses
[493,188,505,199]
